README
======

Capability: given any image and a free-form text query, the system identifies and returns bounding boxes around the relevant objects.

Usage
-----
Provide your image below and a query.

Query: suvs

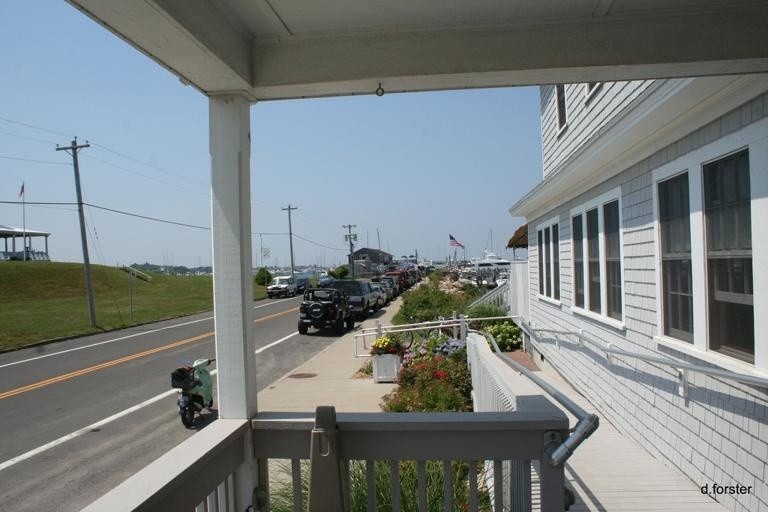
[266,275,312,299]
[298,265,435,336]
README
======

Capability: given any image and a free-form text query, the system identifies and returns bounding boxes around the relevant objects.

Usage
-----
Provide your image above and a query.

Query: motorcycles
[172,358,215,428]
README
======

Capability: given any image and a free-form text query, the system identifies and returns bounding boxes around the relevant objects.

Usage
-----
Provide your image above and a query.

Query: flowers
[368,335,404,354]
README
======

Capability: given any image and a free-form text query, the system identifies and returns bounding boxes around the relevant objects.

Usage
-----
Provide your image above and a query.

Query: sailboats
[449,225,511,277]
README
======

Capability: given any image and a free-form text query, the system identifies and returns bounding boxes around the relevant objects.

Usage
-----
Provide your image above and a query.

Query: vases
[372,353,402,383]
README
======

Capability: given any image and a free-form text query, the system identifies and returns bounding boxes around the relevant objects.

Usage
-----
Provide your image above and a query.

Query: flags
[449,234,464,249]
[19,182,24,197]
